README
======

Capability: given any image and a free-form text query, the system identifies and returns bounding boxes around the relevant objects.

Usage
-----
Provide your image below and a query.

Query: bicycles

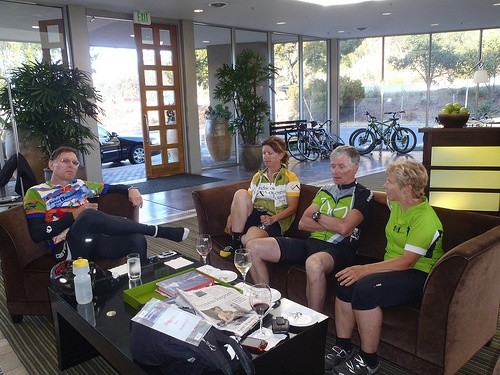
[349,109,417,156]
[288,119,345,162]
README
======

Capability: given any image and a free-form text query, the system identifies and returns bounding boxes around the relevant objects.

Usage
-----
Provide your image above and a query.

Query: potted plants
[205,104,235,161]
[212,49,273,171]
[0,58,105,181]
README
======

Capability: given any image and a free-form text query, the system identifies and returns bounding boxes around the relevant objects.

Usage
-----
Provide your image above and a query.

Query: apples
[440,103,470,114]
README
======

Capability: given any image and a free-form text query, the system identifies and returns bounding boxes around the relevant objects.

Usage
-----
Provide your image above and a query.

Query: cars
[98,126,162,164]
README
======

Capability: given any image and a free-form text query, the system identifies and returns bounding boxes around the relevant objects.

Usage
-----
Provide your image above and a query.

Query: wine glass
[257,198,270,229]
[233,248,252,289]
[248,283,271,339]
[195,234,212,274]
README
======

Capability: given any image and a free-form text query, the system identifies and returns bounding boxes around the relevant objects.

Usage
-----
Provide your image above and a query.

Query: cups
[127,253,142,279]
[128,278,142,289]
[86,188,99,204]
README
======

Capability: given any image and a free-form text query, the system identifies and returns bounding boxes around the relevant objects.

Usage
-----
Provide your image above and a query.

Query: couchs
[189,184,500,375]
[0,192,138,323]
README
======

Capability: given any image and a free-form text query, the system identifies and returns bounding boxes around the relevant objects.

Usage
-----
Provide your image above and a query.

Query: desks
[418,125,500,213]
[46,251,328,375]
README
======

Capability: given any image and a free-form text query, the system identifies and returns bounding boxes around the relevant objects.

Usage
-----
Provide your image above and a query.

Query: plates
[211,270,237,284]
[271,288,281,302]
[281,307,318,327]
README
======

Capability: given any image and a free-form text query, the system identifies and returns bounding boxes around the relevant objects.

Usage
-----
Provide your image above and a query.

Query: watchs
[312,211,322,222]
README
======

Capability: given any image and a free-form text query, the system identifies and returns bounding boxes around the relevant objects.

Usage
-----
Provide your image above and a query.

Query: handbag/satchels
[130,305,255,374]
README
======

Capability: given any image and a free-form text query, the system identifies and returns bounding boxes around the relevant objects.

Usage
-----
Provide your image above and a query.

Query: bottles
[78,302,97,327]
[73,259,92,304]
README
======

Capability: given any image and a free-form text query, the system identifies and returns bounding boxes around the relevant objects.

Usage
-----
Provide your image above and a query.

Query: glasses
[55,158,81,169]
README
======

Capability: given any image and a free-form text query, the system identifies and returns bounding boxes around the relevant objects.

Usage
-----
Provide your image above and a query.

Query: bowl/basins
[438,114,469,128]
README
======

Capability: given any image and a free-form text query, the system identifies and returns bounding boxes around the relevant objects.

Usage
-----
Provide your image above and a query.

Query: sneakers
[325,346,355,371]
[332,352,381,375]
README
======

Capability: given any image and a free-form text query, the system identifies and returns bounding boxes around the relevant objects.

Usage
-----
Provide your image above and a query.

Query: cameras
[241,337,268,352]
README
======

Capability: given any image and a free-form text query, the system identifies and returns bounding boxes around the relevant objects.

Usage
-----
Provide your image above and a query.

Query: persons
[245,145,374,315]
[220,137,301,256]
[22,147,190,263]
[324,159,444,375]
